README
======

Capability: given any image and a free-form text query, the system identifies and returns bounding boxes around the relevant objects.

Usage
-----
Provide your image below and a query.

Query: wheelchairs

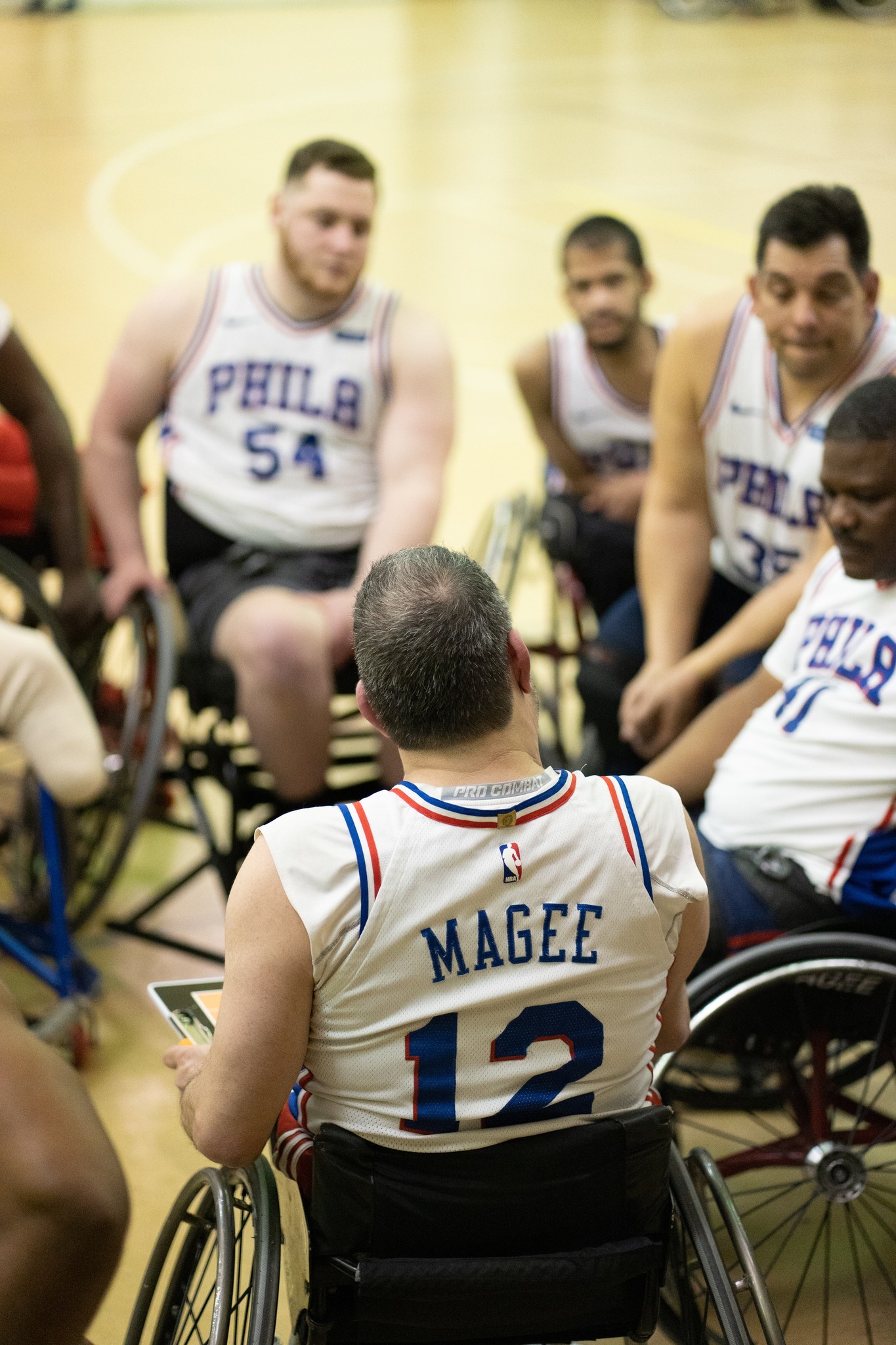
[127,1148,790,1345]
[1,421,630,1072]
[651,913,896,1345]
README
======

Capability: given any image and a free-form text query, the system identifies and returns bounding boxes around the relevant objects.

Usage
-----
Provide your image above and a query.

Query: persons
[0,986,131,1345]
[166,544,713,1193]
[1,294,114,821]
[83,138,457,843]
[507,220,690,627]
[610,373,896,1005]
[570,183,894,800]
